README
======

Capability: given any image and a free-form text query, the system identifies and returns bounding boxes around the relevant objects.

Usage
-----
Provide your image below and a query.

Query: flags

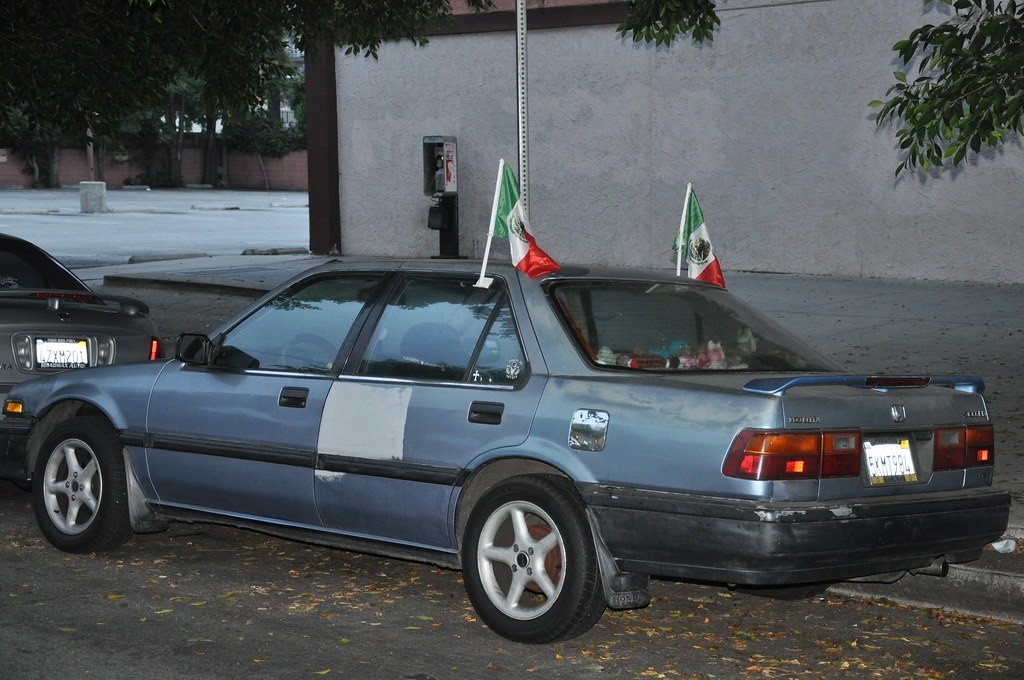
[673,187,724,286]
[490,163,559,273]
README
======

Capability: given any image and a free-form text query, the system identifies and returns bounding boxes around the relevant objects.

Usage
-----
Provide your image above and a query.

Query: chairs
[401,323,464,364]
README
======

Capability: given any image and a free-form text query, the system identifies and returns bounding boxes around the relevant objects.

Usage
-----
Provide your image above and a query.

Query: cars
[0,233,167,419]
[1,257,1013,645]
